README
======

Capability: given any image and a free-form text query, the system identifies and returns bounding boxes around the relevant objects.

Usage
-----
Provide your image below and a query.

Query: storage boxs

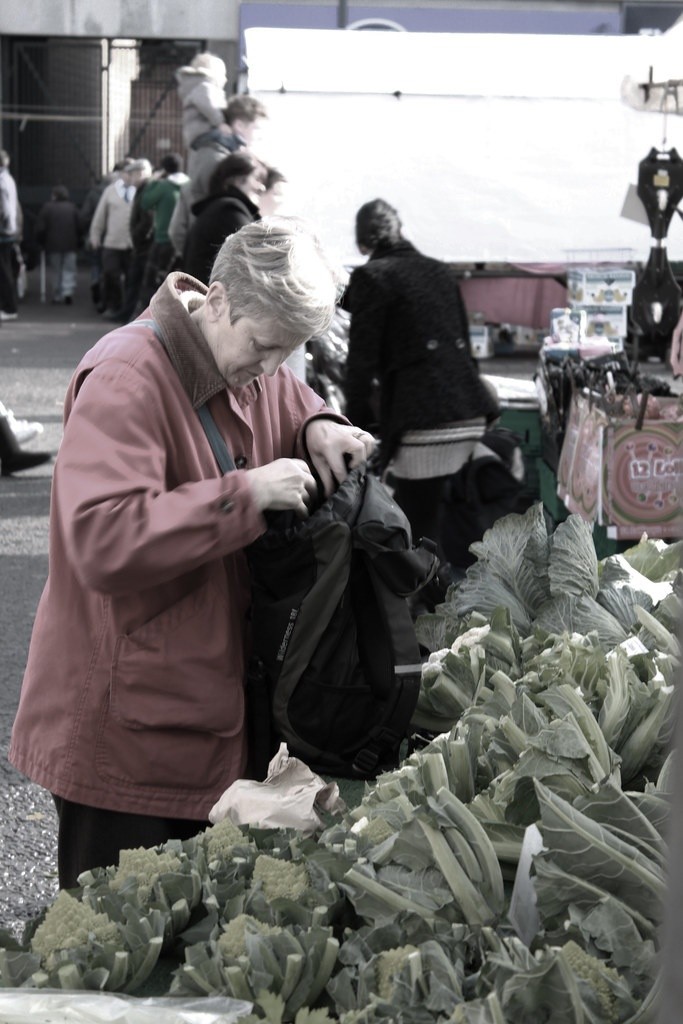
[533,364,683,540]
[551,305,626,338]
[567,268,635,304]
[540,343,614,361]
[540,336,623,352]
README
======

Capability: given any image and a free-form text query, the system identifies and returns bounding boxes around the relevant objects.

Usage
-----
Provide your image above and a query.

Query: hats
[124,159,150,171]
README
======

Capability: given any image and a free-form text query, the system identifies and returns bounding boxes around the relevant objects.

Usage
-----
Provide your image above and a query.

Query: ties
[124,187,129,203]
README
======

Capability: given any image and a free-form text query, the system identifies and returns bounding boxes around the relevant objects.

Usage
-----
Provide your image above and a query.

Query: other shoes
[0,310,18,321]
[0,413,51,476]
[0,401,44,444]
[64,293,74,307]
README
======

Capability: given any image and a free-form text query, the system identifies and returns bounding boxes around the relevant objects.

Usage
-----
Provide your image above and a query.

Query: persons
[0,52,501,618]
[10,214,379,891]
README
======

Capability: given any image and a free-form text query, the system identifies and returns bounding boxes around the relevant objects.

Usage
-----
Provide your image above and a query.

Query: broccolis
[0,614,683,1022]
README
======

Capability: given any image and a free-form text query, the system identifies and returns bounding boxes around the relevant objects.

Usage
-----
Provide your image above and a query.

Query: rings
[355,430,367,438]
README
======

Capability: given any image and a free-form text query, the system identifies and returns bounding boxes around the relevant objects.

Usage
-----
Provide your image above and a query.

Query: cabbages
[413,501,683,672]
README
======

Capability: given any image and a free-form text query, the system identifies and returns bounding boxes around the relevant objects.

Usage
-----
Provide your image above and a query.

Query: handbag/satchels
[556,356,683,540]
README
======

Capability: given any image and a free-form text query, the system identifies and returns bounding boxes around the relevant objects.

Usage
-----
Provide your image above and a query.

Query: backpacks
[242,462,438,780]
